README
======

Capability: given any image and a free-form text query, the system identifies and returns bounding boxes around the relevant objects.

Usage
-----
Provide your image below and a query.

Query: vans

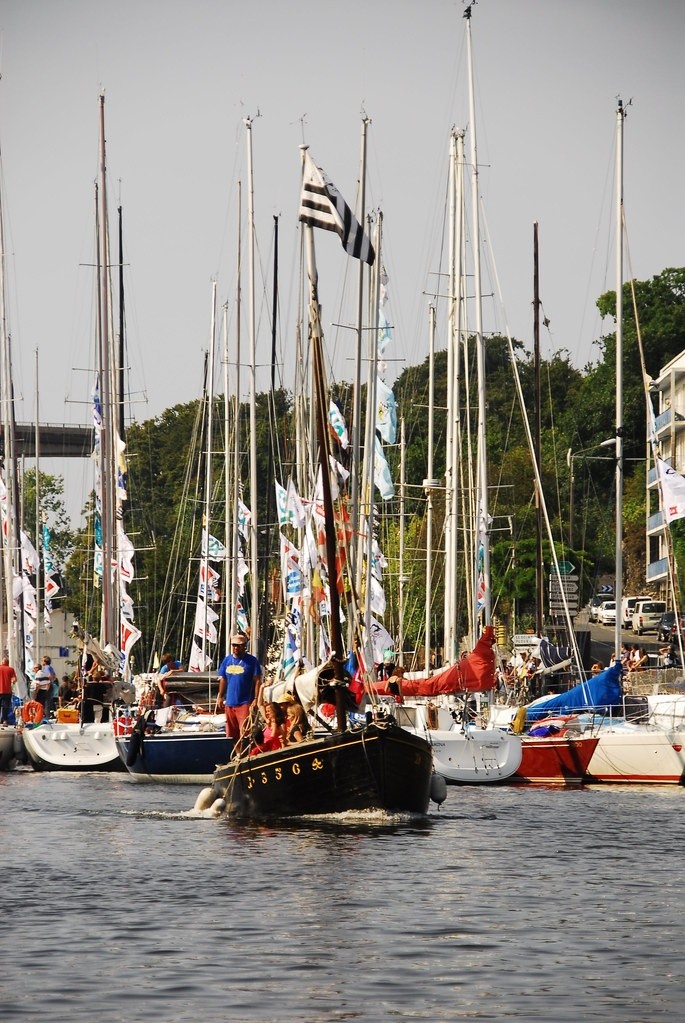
[629,601,667,636]
[622,596,653,629]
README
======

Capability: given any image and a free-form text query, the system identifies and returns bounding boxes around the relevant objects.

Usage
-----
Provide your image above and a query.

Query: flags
[1,478,60,678]
[299,154,376,266]
[477,502,493,612]
[658,457,685,525]
[190,268,396,683]
[92,381,142,675]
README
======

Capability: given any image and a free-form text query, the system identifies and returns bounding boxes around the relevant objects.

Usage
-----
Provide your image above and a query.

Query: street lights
[570,438,615,562]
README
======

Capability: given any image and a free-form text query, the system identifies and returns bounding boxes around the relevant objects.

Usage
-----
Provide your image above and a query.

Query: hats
[231,635,246,644]
[520,652,526,654]
[276,694,295,705]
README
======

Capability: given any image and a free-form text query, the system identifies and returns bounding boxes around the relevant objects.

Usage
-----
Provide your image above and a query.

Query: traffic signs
[549,574,579,581]
[549,600,576,601]
[549,581,579,593]
[549,609,579,617]
[549,561,576,574]
[549,601,579,608]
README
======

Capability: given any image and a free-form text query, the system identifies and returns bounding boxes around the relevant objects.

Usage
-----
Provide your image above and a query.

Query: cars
[658,611,685,642]
[670,615,685,643]
[597,601,615,626]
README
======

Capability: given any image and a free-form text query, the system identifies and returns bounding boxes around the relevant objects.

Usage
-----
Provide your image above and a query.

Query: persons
[377,658,395,681]
[249,679,311,756]
[385,666,406,705]
[659,645,679,667]
[158,654,193,714]
[496,651,568,705]
[217,635,264,755]
[0,658,18,723]
[34,656,108,717]
[610,644,650,672]
[591,663,603,677]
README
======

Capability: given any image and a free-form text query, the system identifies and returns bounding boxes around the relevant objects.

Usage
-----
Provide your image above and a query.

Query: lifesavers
[136,691,152,715]
[22,700,43,724]
[514,708,527,733]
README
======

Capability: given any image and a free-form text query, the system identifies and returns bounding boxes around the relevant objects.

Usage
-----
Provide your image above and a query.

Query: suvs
[589,597,614,622]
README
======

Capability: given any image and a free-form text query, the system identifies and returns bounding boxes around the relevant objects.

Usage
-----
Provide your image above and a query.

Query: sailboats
[194,142,446,820]
[2,0,685,790]
